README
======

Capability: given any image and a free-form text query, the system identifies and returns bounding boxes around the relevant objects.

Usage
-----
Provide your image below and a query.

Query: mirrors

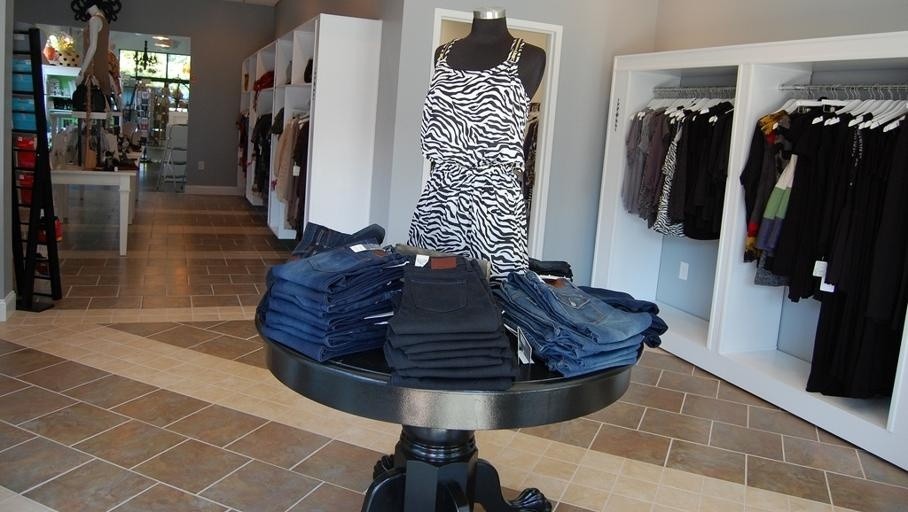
[422,8,563,262]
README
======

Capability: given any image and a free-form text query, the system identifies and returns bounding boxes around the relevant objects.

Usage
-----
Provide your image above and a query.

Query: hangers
[292,102,310,124]
[772,83,908,132]
[526,103,540,124]
[637,87,735,123]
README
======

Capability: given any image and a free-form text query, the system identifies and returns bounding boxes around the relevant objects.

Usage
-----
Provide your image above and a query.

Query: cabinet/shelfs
[590,31,908,472]
[41,63,81,149]
[237,14,383,241]
[50,110,146,256]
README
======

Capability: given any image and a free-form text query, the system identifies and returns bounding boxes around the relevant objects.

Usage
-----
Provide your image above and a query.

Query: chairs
[157,124,188,192]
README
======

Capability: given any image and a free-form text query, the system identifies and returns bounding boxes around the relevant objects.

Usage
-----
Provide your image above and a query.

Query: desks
[255,313,631,512]
